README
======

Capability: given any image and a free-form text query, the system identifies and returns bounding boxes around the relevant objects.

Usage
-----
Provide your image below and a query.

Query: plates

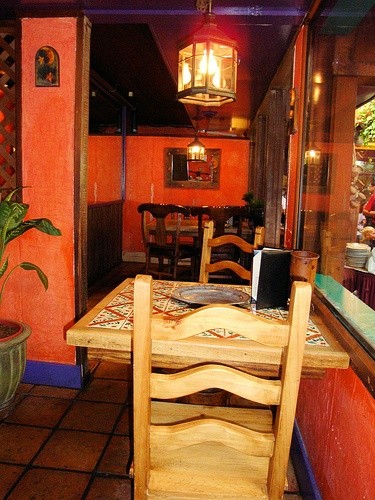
[344,243,371,268]
[172,285,251,305]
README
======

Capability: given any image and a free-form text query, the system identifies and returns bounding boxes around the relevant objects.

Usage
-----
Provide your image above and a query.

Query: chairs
[129,201,313,500]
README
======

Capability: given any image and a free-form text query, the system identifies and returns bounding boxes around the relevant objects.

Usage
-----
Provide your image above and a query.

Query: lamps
[186,129,207,163]
[176,0,241,106]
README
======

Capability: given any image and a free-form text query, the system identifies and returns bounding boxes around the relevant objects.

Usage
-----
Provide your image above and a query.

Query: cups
[289,251,320,292]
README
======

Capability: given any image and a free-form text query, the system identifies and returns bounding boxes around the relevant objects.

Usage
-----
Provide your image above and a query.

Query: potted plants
[0,186,62,419]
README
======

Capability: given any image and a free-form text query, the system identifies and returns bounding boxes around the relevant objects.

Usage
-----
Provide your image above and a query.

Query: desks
[342,265,375,311]
[146,220,253,281]
[66,277,349,492]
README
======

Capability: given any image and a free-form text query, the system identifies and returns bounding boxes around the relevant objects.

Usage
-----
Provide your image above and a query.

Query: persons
[357,182,375,230]
[361,226,375,249]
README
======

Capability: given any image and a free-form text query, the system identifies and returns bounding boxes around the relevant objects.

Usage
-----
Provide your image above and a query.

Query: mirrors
[165,148,221,188]
